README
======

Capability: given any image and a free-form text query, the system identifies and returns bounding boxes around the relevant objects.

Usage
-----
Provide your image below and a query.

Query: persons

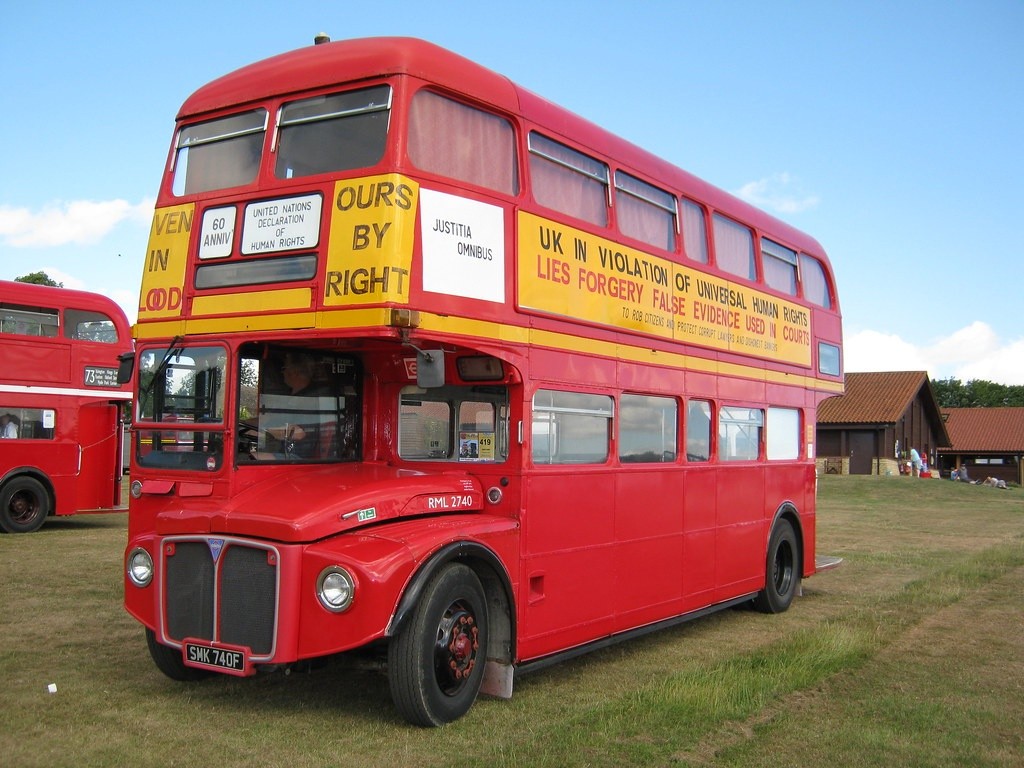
[907,446,920,477]
[920,450,926,471]
[241,354,328,460]
[954,464,976,484]
[950,468,958,480]
[984,476,1006,489]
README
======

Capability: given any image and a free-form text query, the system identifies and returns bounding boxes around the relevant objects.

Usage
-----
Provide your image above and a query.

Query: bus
[0,279,135,533]
[116,33,846,728]
